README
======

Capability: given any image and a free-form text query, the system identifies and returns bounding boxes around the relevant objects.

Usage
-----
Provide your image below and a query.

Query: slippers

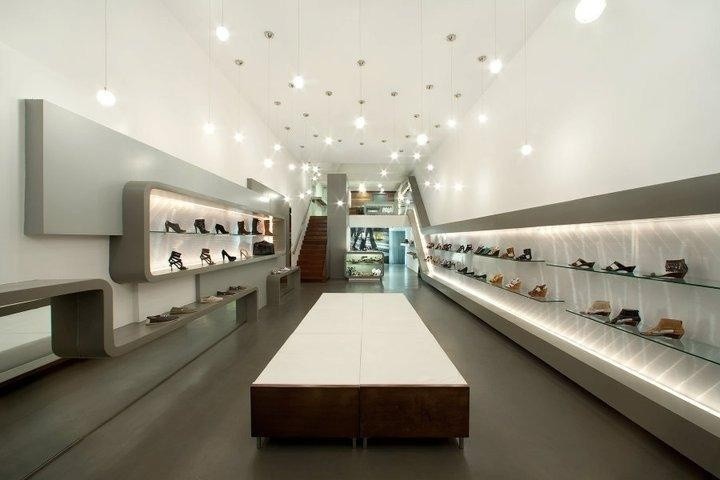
[601,261,637,273]
[568,257,595,268]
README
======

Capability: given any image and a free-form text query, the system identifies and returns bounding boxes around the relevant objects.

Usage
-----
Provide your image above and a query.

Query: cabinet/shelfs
[400,243,720,365]
[0,181,301,358]
[344,252,384,279]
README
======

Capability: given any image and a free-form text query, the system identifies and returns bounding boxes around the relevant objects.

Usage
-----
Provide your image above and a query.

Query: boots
[238,221,250,235]
[252,218,262,234]
[264,219,273,235]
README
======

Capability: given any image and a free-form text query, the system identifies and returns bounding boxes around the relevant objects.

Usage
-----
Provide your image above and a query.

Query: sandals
[641,258,689,278]
[604,308,642,326]
[426,241,532,291]
[580,300,612,315]
[640,318,685,339]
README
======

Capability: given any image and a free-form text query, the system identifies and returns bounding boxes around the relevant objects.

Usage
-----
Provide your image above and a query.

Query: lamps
[96,0,607,207]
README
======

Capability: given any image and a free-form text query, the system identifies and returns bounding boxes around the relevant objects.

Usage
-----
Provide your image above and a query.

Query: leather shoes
[200,296,223,303]
[229,286,248,291]
[146,314,179,324]
[217,290,235,297]
[169,307,197,314]
[280,266,291,271]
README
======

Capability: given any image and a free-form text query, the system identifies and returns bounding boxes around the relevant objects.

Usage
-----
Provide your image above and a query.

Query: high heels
[528,283,547,297]
[240,248,251,259]
[165,219,237,274]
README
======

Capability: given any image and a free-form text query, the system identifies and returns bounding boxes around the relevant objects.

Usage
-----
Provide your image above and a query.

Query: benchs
[250,292,470,449]
